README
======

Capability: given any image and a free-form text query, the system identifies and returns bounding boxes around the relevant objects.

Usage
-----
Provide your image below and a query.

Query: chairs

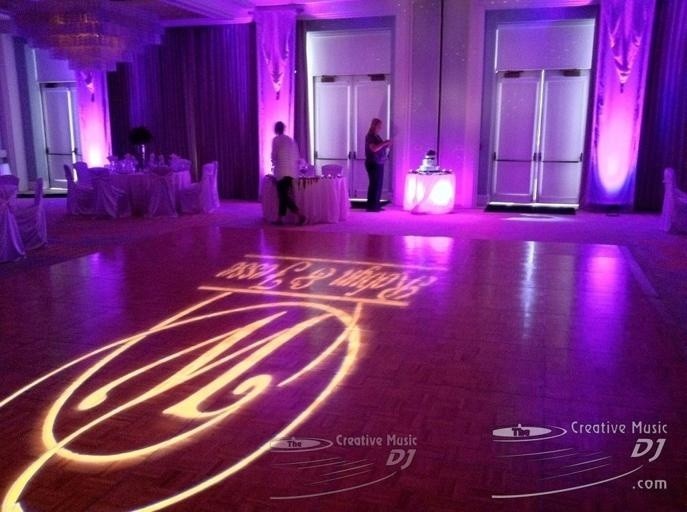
[0,152,220,264]
[661,165,687,234]
[300,164,343,178]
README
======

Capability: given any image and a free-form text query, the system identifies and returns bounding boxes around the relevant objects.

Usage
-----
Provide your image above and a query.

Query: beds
[263,173,347,226]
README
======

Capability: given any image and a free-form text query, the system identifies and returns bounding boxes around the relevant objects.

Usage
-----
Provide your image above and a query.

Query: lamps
[7,0,163,101]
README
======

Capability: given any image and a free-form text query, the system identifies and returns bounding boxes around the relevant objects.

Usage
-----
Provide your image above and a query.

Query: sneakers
[367,207,382,210]
[271,214,308,226]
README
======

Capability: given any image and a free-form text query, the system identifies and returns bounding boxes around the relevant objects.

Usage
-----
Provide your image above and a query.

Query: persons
[363,118,390,211]
[271,120,307,226]
